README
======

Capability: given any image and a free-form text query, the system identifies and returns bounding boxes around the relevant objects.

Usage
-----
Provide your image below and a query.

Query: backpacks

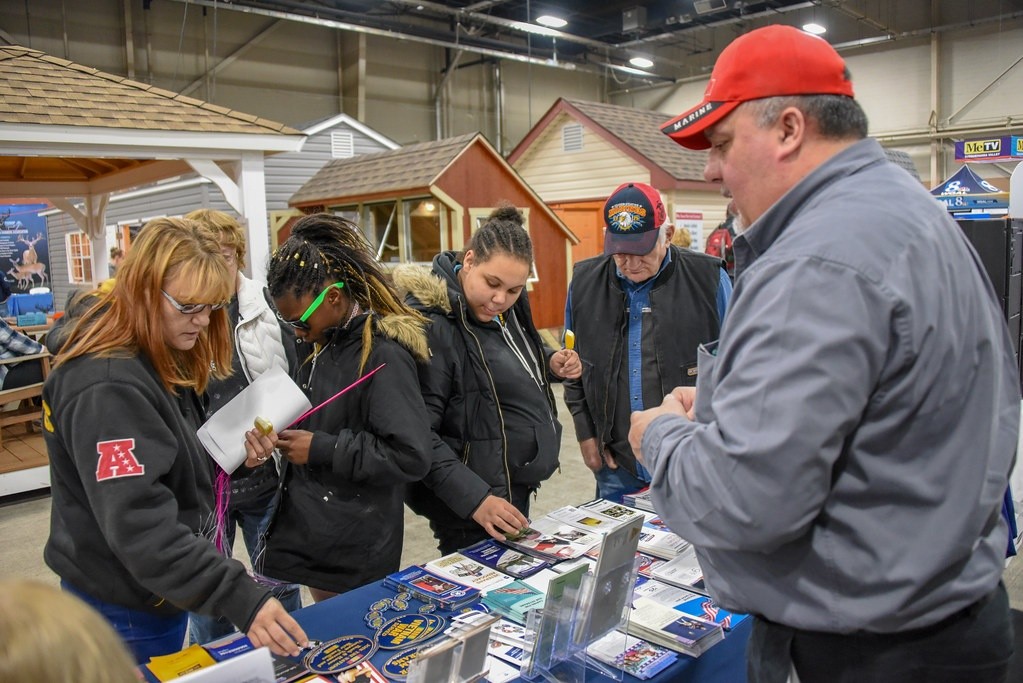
[706,223,735,268]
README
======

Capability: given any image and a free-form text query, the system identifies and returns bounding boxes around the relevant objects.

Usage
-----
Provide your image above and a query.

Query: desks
[4,315,54,332]
[136,486,753,683]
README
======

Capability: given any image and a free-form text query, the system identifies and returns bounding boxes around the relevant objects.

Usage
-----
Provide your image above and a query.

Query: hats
[93,278,117,302]
[603,182,666,256]
[659,25,854,152]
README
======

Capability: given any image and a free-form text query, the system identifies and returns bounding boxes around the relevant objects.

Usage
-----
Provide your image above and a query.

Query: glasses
[158,288,226,315]
[276,282,345,331]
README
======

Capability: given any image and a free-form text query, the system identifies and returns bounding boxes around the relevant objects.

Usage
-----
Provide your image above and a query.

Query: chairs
[0,354,50,433]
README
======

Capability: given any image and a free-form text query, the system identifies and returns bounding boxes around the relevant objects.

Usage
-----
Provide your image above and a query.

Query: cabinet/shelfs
[955,218,1023,398]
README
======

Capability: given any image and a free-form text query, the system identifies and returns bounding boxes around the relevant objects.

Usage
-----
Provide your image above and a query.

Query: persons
[0,207,432,683]
[402,202,585,544]
[625,21,1023,683]
[560,181,735,508]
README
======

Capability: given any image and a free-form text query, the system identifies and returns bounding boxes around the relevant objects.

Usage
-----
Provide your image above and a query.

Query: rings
[258,457,268,461]
